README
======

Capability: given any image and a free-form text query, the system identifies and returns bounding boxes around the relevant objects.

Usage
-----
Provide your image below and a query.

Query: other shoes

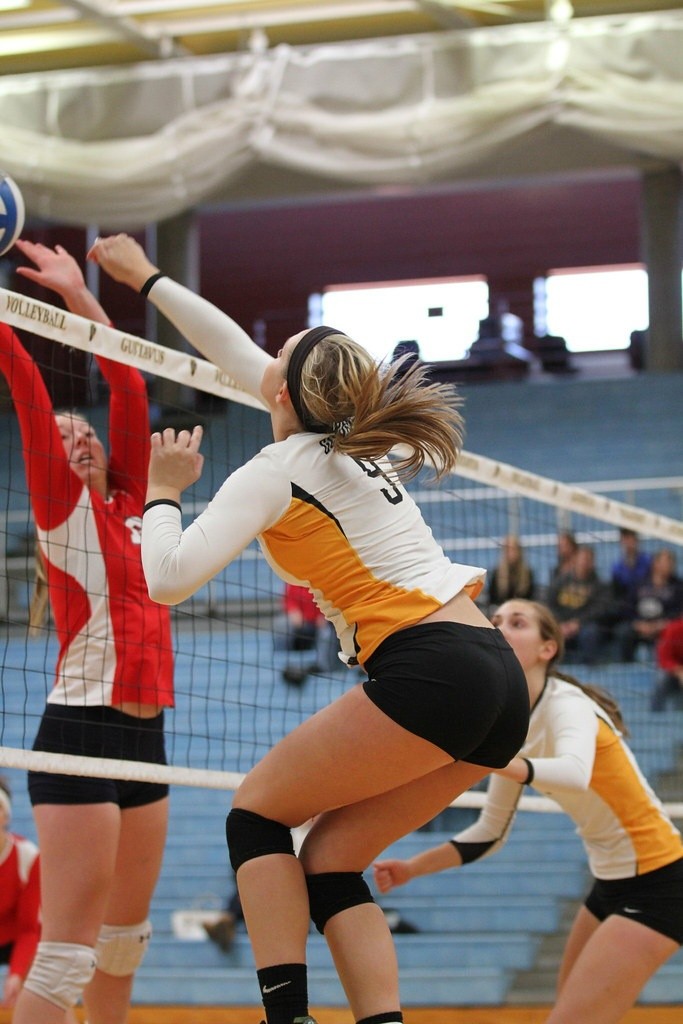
[200,916,237,953]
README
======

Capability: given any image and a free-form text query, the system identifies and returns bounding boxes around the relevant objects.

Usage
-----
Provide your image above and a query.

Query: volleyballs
[0,166,27,256]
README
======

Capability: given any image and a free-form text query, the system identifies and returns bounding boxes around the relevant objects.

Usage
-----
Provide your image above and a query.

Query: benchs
[0,372,683,1008]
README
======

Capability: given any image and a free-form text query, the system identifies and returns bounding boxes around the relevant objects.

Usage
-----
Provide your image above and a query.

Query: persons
[0,240,176,1024]
[203,814,316,955]
[374,599,683,1024]
[86,233,531,1024]
[546,528,683,713]
[0,776,78,1024]
[272,581,344,685]
[486,532,536,619]
